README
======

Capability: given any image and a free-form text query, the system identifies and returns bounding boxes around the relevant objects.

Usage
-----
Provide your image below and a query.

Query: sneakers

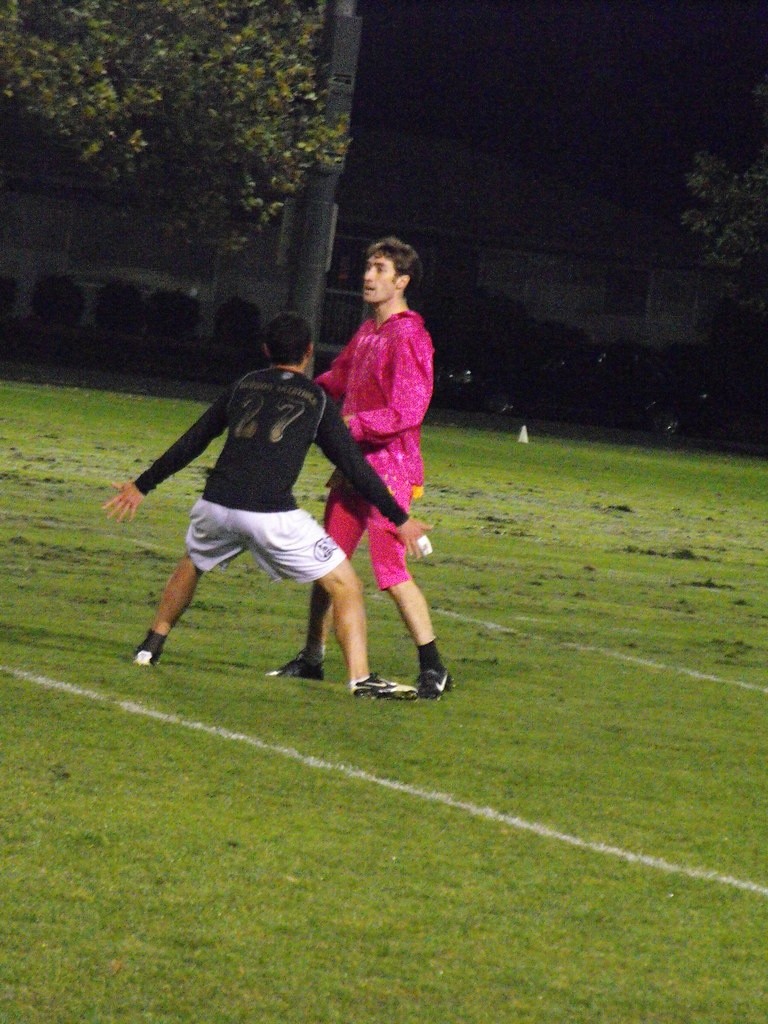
[131,638,165,668]
[263,646,326,680]
[349,671,419,701]
[416,664,456,702]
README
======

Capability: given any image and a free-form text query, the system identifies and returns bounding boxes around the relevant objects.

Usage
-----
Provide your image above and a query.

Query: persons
[266,238,456,700]
[102,312,434,700]
